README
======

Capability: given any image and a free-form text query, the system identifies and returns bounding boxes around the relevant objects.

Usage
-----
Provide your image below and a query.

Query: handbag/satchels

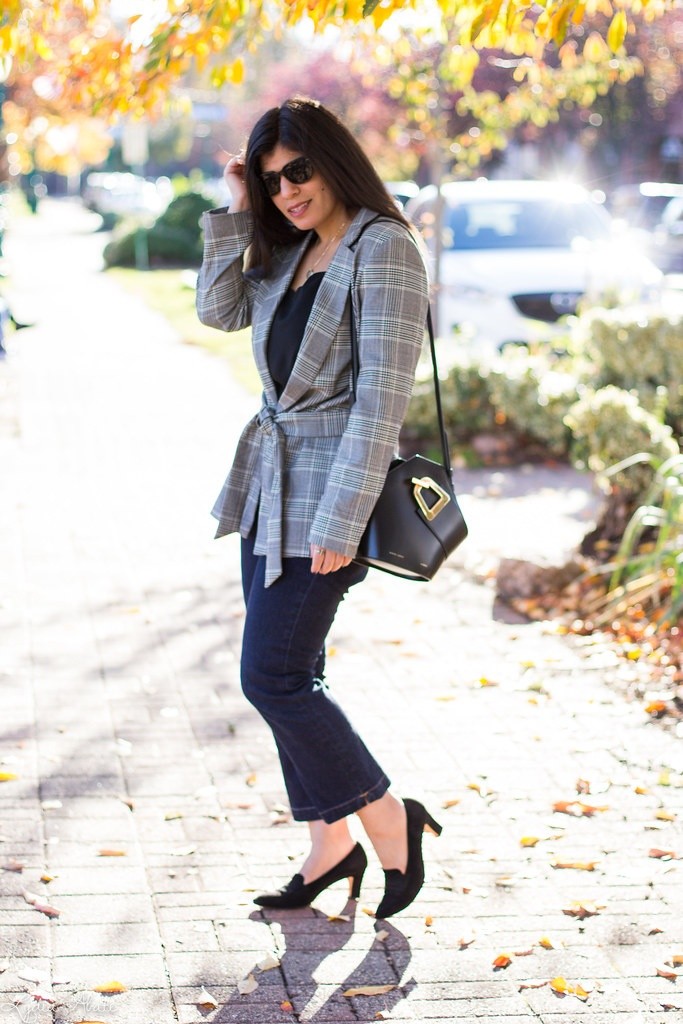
[352,453,469,580]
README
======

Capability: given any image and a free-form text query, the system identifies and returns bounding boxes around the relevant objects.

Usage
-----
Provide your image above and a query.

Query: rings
[315,548,324,554]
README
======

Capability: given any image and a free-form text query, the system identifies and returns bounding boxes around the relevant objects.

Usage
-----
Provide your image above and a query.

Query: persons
[195,101,443,920]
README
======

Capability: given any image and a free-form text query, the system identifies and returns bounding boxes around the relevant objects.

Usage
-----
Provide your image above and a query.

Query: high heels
[375,799,442,919]
[253,842,368,909]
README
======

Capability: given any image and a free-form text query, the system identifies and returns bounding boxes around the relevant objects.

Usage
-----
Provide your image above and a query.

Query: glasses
[259,154,314,196]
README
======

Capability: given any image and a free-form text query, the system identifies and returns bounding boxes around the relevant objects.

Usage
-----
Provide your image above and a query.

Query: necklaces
[305,222,345,278]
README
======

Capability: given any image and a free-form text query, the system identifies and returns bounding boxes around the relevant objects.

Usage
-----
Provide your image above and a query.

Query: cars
[379,175,683,350]
